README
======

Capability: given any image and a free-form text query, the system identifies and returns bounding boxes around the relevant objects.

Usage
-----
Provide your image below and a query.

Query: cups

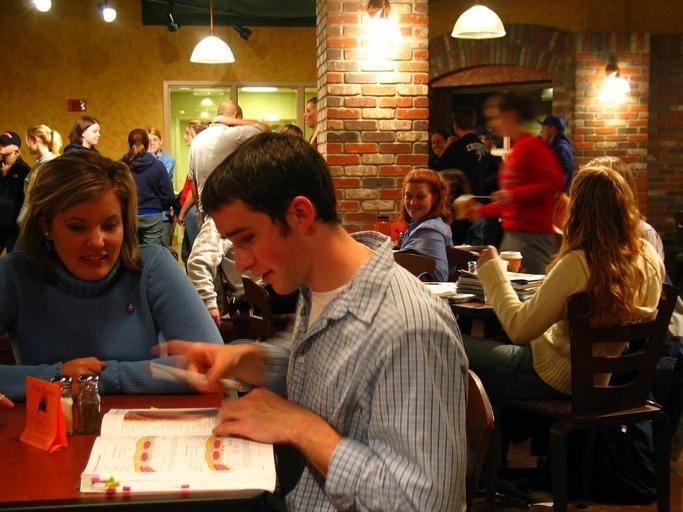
[499,250,524,273]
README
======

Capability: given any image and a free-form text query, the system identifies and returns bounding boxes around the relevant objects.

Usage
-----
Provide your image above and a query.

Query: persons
[454,166,665,512]
[398,165,456,283]
[153,131,468,511]
[432,87,574,273]
[583,155,683,471]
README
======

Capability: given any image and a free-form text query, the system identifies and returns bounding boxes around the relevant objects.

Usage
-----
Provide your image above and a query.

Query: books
[456,267,546,302]
[80,408,279,503]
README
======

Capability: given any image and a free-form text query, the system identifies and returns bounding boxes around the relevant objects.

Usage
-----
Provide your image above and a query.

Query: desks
[427,281,505,342]
[0,383,283,511]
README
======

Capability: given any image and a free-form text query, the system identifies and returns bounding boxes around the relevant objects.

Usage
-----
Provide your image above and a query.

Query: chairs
[388,246,432,284]
[461,360,501,509]
[445,243,480,285]
[233,275,288,339]
[491,277,681,511]
[209,253,265,344]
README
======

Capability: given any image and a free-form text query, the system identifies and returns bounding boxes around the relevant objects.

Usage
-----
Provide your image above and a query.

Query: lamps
[188,1,234,69]
[360,1,405,80]
[601,56,630,114]
[228,17,253,46]
[162,7,183,33]
[450,1,511,45]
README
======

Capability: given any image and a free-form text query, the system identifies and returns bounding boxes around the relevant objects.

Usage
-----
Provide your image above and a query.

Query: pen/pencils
[148,361,246,395]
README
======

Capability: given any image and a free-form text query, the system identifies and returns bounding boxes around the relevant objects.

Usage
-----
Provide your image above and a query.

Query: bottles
[73,374,102,434]
[48,375,73,436]
[467,261,477,274]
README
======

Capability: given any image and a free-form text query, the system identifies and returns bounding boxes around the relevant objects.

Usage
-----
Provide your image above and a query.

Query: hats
[0,131,22,148]
[535,112,568,131]
